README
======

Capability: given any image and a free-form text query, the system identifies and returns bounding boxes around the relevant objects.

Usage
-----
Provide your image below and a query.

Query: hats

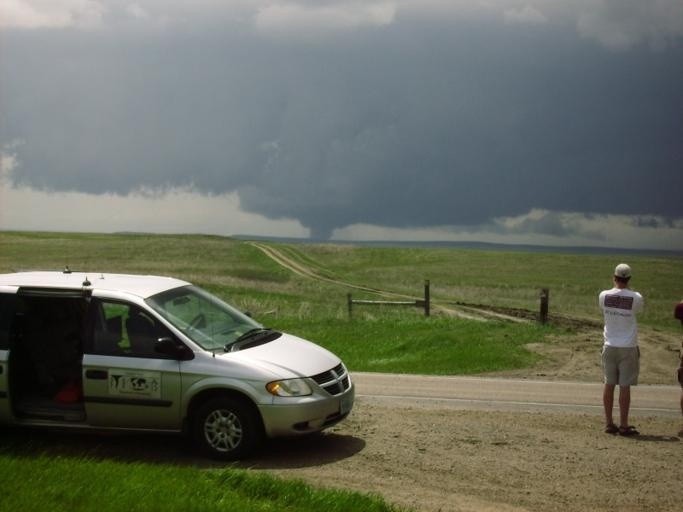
[615,264,632,278]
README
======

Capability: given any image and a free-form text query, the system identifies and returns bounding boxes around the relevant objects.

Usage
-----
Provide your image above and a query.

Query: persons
[598,263,644,435]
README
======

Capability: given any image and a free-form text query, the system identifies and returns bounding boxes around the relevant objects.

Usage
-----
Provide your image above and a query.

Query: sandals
[606,424,640,435]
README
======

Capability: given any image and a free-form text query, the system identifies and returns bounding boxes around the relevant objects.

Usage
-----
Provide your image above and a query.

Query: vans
[0,265,356,463]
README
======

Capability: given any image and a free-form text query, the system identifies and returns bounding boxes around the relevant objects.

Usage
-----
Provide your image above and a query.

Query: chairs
[125,307,162,356]
